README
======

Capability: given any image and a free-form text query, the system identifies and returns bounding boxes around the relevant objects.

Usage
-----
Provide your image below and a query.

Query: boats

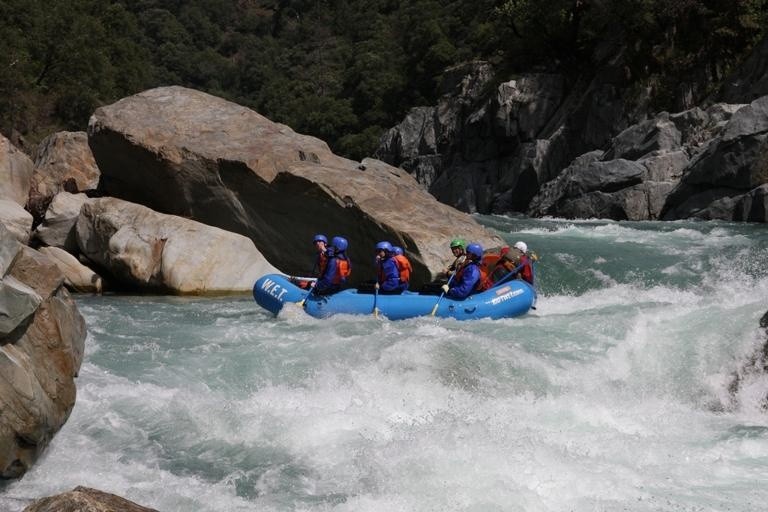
[251,272,534,321]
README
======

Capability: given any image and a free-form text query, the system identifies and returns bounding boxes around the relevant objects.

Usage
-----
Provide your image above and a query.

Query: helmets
[466,243,482,256]
[513,242,527,254]
[450,240,465,250]
[377,242,393,251]
[392,247,403,254]
[313,235,327,243]
[333,237,348,250]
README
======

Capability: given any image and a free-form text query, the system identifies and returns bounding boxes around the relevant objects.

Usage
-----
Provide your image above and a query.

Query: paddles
[431,269,456,316]
[372,256,381,317]
[288,282,315,318]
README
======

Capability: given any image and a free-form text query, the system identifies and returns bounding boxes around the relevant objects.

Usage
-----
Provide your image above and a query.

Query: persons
[442,238,467,277]
[313,234,329,274]
[490,241,538,287]
[374,240,402,295]
[439,243,491,300]
[313,236,353,297]
[391,245,413,295]
